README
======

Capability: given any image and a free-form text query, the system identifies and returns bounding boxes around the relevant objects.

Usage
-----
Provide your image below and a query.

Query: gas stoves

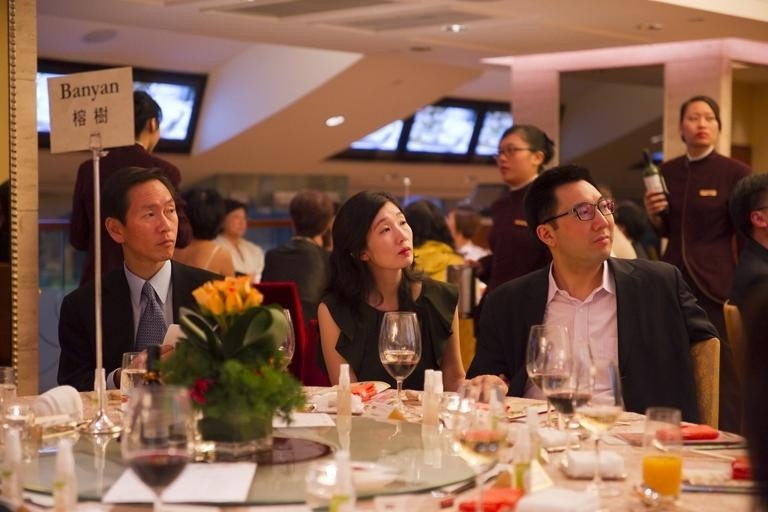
[0,366,39,486]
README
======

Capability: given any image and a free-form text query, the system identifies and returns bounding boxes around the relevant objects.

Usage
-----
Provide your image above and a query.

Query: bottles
[640,145,673,217]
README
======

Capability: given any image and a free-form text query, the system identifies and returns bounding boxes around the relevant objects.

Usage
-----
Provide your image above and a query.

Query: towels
[459,488,520,512]
[346,382,377,404]
[731,453,750,478]
[656,424,719,444]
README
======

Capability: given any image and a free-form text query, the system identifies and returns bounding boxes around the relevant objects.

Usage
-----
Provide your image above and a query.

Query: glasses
[536,194,619,224]
[494,144,534,161]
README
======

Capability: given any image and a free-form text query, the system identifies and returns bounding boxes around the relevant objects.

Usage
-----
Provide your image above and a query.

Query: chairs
[245,280,331,386]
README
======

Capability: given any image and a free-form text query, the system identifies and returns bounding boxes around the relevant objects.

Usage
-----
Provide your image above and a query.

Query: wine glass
[526,325,572,430]
[451,374,509,512]
[543,337,596,469]
[378,311,422,409]
[122,352,148,389]
[277,309,294,371]
[572,355,624,498]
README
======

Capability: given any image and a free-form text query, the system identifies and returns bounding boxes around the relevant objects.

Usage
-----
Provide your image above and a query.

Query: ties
[134,281,169,356]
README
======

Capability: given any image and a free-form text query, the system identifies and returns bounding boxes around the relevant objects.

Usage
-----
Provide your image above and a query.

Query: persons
[474,123,556,319]
[643,94,756,349]
[214,199,268,282]
[261,192,336,372]
[457,164,743,424]
[56,165,229,391]
[317,190,513,393]
[323,201,345,252]
[612,196,650,259]
[68,90,193,289]
[729,171,768,358]
[399,199,477,374]
[171,187,234,278]
[443,204,494,264]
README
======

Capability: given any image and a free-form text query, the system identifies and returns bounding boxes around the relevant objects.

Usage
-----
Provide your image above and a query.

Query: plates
[681,436,742,444]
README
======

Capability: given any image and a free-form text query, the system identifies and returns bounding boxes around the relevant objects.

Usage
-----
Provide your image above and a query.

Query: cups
[120,385,196,512]
[640,408,682,503]
